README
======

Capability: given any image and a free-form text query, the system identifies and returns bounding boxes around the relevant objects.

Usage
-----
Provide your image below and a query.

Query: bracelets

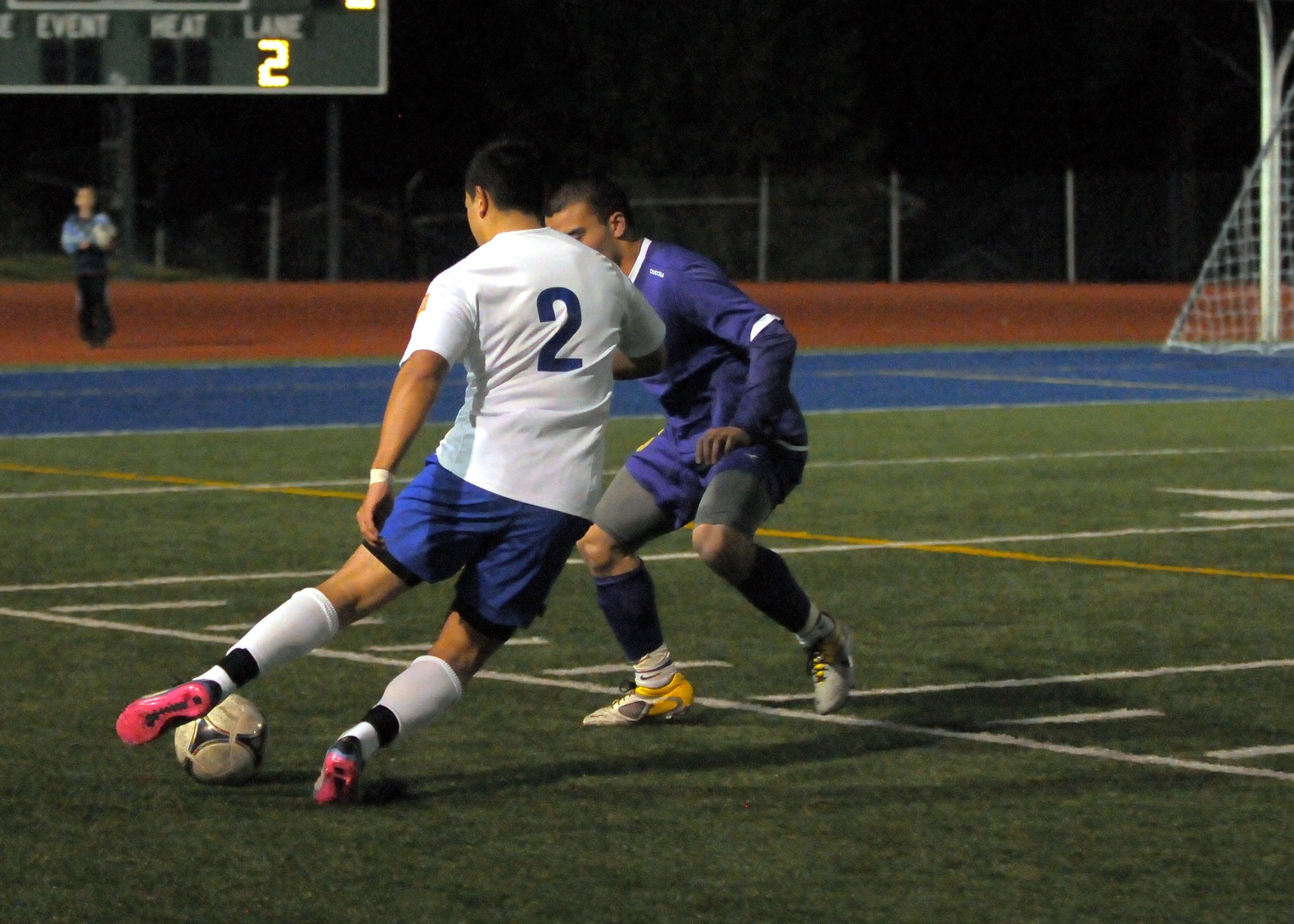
[369,468,396,486]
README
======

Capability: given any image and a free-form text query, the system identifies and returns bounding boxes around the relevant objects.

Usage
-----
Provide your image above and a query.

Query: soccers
[175,693,267,784]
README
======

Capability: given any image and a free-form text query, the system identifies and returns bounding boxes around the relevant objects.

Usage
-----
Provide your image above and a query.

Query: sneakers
[314,735,366,804]
[582,673,695,727]
[116,679,222,745]
[798,610,849,716]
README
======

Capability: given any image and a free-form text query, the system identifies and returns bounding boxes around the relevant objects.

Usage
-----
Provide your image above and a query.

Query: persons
[544,171,853,726]
[61,184,116,349]
[116,143,668,803]
